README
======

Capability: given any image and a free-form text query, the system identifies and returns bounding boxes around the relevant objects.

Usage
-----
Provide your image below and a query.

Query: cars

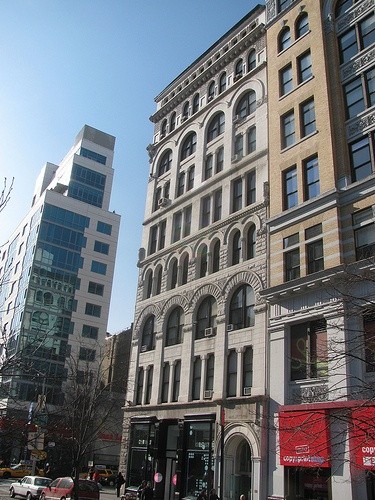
[96,468,116,487]
[0,463,46,479]
[9,476,53,500]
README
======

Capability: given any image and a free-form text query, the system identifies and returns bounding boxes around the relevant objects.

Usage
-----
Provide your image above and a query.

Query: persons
[196,489,207,500]
[114,471,124,498]
[43,463,51,478]
[89,468,94,479]
[136,479,155,500]
[240,494,245,500]
[209,489,219,500]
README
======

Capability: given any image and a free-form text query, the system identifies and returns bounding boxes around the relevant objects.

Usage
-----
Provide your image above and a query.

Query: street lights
[19,361,46,476]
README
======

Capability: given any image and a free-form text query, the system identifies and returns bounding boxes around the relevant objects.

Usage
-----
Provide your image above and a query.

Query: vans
[39,477,100,500]
